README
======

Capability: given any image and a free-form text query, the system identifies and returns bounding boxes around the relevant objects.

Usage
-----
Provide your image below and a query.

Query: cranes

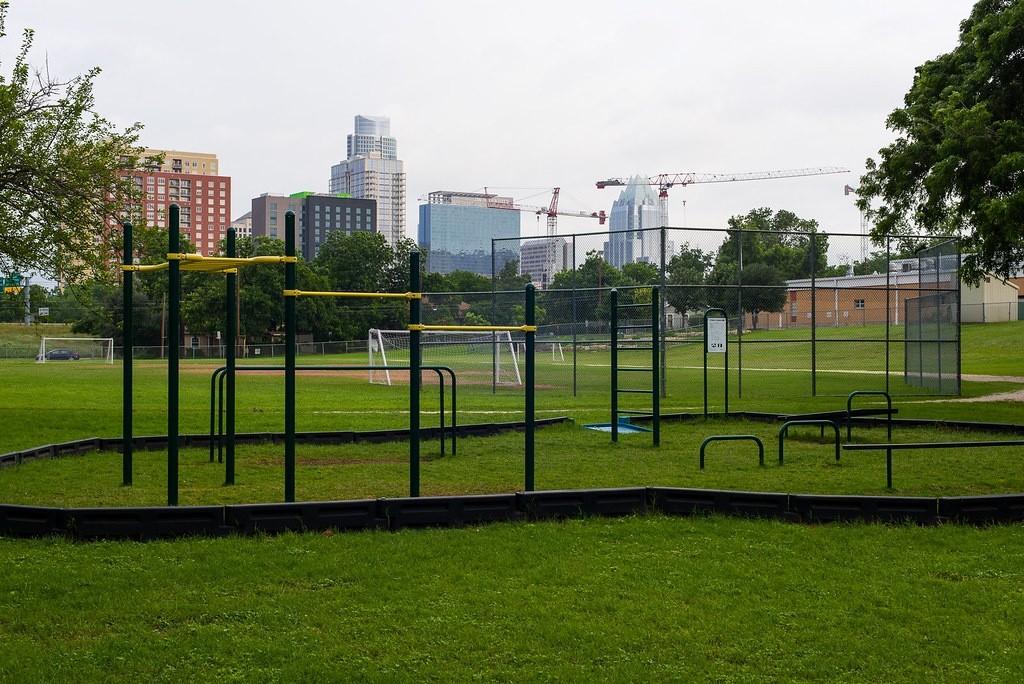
[595,167,851,239]
[478,185,613,286]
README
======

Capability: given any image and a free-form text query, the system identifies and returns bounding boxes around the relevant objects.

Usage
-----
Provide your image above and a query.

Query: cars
[35,349,80,361]
